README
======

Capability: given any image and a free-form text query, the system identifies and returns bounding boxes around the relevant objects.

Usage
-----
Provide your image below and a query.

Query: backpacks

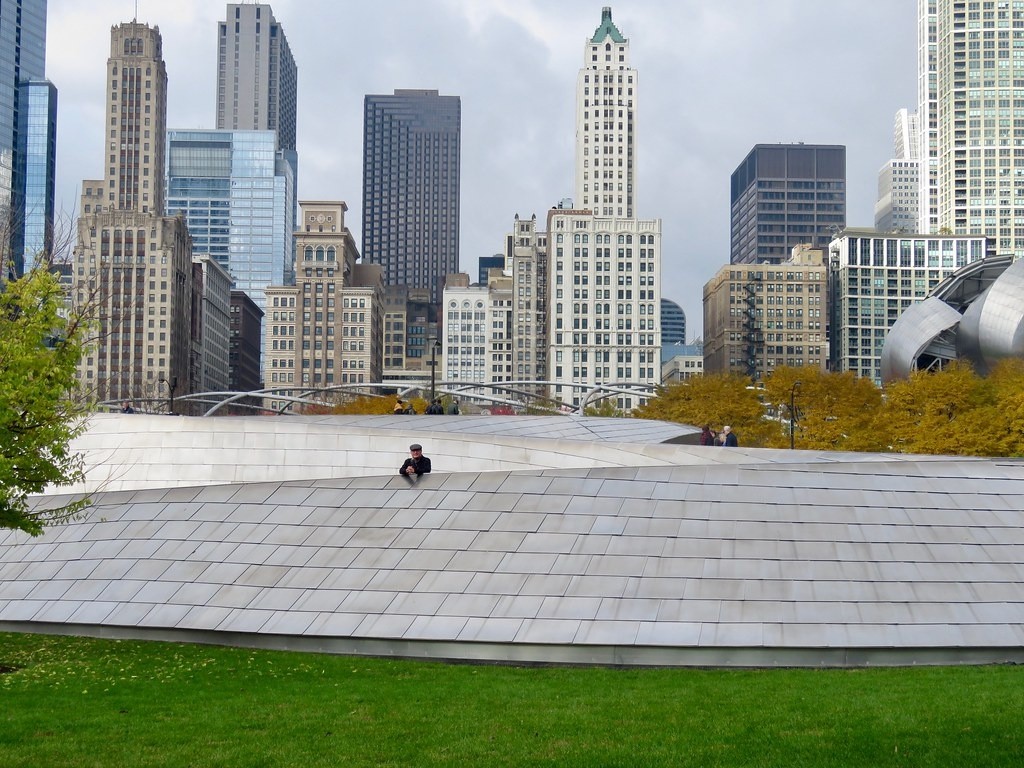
[425,405,434,415]
[404,409,410,415]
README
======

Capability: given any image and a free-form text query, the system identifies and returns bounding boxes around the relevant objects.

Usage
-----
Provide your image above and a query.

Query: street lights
[791,380,802,449]
[157,378,173,415]
[431,340,442,406]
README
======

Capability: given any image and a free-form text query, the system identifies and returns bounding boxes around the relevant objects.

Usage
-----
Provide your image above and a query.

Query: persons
[448,399,459,415]
[403,404,417,414]
[700,425,738,447]
[399,444,431,477]
[394,400,403,414]
[424,399,444,415]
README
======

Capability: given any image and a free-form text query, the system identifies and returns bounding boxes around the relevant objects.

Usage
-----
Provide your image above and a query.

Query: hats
[702,426,709,431]
[431,399,436,404]
[410,444,422,451]
